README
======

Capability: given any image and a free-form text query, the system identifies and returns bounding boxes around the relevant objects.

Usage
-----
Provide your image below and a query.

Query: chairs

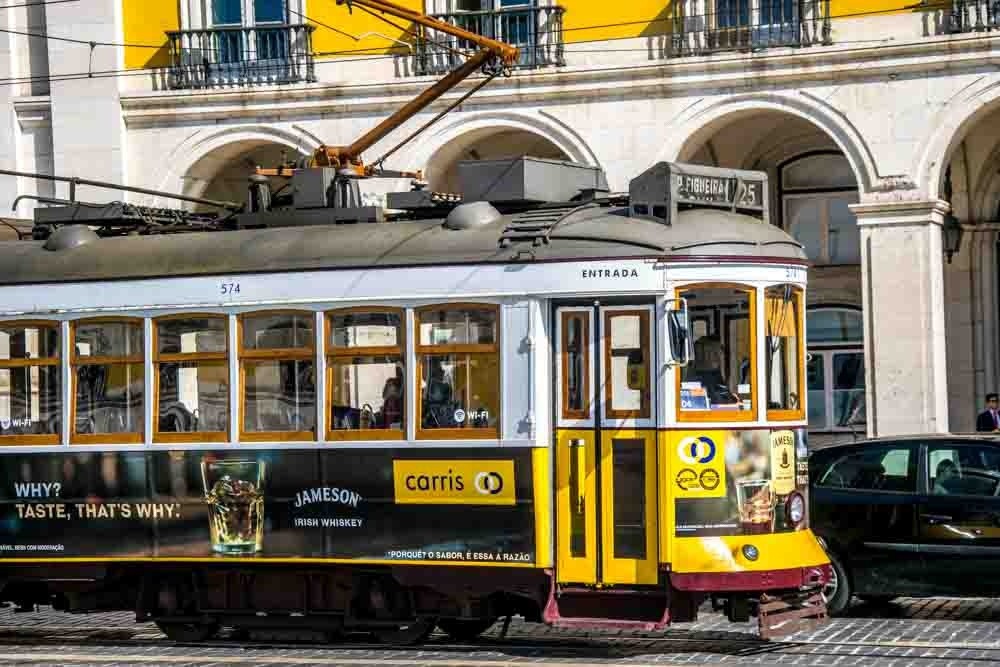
[429,382,464,425]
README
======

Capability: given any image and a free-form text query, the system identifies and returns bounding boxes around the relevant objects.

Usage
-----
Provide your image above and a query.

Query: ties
[994,411,998,426]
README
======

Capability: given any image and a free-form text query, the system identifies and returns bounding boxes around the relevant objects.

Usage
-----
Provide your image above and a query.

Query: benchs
[835,460,888,487]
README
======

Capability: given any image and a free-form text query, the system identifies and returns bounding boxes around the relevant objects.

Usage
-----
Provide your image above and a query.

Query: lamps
[942,164,964,263]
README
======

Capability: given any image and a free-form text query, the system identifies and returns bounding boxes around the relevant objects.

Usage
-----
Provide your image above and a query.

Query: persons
[422,358,445,385]
[860,464,887,490]
[382,378,400,424]
[976,393,1000,432]
[685,334,738,403]
[933,458,960,495]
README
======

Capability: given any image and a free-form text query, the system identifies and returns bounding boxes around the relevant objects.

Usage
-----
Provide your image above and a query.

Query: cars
[763,432,1000,617]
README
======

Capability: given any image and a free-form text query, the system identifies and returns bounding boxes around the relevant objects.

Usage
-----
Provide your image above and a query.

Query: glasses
[989,399,999,403]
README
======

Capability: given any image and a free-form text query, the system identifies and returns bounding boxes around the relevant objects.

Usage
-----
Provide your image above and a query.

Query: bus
[0,146,830,644]
